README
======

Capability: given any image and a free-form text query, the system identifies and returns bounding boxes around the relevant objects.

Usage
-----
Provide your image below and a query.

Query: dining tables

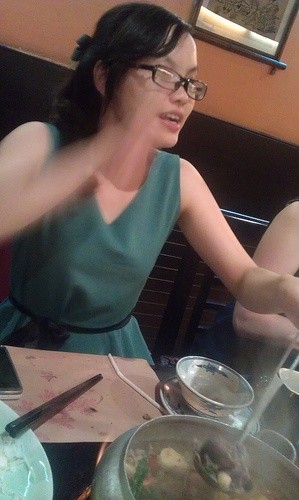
[0,358,299,500]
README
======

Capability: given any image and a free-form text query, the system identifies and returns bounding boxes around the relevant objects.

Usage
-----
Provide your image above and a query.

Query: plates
[156,375,260,435]
[0,399,54,499]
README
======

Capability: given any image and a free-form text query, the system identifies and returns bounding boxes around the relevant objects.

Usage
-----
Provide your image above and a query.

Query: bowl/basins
[176,355,255,423]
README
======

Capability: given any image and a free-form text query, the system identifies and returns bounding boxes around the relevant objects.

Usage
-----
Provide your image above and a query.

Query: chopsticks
[5,373,103,436]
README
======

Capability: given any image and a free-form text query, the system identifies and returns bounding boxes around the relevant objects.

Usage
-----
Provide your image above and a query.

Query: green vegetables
[129,456,220,500]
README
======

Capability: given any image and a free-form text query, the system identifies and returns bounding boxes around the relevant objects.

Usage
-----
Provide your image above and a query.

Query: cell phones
[0,344,23,400]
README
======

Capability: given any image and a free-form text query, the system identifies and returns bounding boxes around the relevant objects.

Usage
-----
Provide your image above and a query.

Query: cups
[253,429,296,465]
[262,367,299,431]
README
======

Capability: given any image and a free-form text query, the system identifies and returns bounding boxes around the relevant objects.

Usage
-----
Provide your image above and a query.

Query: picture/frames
[188,0,299,75]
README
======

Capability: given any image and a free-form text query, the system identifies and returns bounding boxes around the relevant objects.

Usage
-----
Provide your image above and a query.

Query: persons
[0,3,299,366]
[232,200,299,351]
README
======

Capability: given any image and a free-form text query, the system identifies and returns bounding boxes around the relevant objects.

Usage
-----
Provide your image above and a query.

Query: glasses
[130,64,209,101]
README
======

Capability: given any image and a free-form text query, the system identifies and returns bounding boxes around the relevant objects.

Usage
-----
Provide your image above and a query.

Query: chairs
[152,209,271,356]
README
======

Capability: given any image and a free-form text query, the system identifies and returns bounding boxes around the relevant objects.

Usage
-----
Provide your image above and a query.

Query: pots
[89,415,299,500]
